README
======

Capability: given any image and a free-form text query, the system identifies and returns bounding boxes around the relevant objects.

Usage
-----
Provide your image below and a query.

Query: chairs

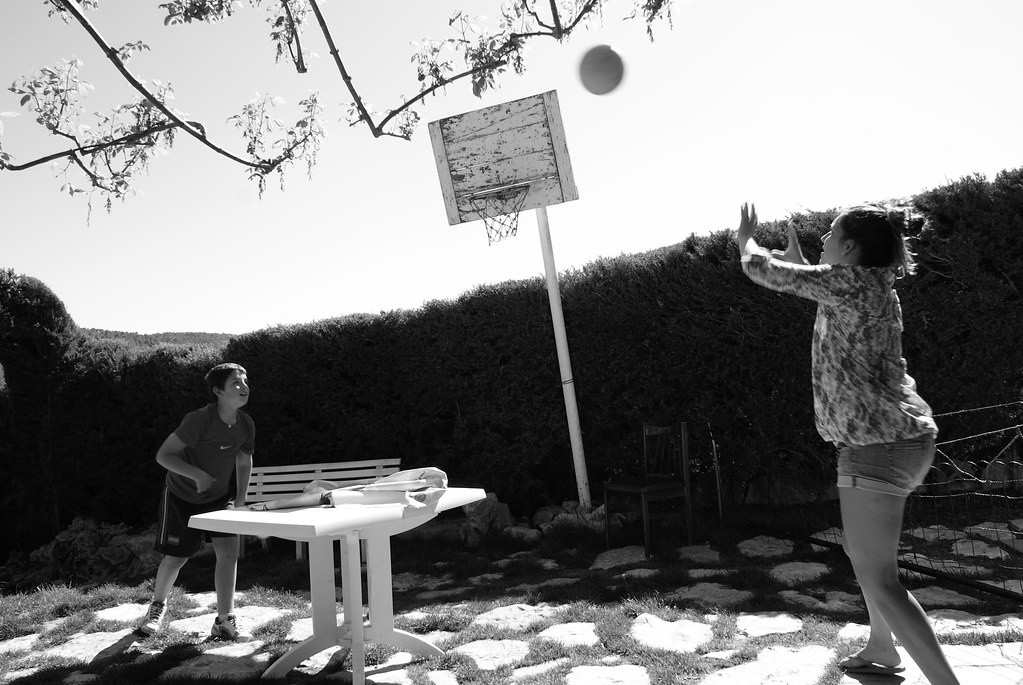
[604,421,695,558]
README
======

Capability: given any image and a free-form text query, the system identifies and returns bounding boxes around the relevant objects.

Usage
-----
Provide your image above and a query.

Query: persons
[737,201,960,685]
[140,363,255,643]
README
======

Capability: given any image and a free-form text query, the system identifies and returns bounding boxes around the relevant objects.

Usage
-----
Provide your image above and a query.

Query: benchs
[237,458,401,562]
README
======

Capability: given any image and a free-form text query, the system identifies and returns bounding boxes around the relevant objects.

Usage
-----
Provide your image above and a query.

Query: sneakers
[141,601,167,635]
[211,613,247,642]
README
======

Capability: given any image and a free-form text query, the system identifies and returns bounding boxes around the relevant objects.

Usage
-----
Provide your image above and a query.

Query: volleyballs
[578,43,627,96]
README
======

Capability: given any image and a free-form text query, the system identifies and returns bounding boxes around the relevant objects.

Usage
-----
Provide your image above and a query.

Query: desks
[188,487,487,685]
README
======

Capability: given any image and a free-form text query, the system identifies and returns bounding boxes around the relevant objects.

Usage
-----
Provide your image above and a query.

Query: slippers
[838,655,905,673]
